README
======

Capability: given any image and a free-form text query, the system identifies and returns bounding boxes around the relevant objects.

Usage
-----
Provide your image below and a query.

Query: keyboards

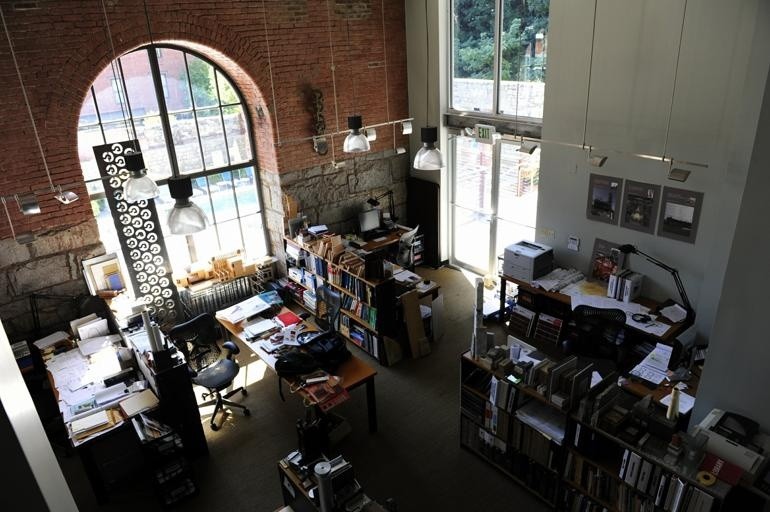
[363,231,386,242]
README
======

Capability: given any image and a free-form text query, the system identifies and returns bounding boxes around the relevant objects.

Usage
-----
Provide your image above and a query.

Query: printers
[502,239,554,285]
[692,406,770,478]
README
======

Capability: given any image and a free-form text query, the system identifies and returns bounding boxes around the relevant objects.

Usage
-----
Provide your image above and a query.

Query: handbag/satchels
[275,331,347,377]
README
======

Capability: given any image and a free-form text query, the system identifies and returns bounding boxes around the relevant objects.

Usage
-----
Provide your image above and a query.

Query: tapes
[696,471,716,486]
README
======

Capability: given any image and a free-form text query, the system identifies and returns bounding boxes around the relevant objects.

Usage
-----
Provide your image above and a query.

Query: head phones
[631,313,652,323]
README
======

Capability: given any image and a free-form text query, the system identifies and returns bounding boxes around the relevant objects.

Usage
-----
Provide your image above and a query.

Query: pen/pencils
[645,323,655,329]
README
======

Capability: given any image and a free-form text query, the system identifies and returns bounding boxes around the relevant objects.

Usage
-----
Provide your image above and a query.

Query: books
[211,223,433,365]
[607,268,644,305]
[82,252,126,296]
[10,313,186,452]
[296,367,352,413]
[462,305,743,511]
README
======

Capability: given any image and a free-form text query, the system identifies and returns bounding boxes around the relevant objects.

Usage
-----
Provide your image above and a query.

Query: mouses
[396,232,402,237]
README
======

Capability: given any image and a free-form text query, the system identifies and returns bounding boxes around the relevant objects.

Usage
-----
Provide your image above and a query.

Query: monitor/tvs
[356,209,382,235]
[668,324,699,380]
